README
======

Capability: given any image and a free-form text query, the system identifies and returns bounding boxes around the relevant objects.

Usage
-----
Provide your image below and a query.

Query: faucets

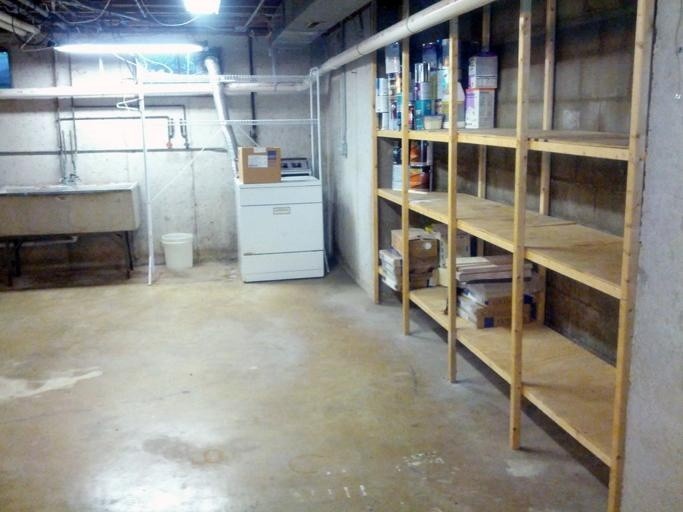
[57,173,83,185]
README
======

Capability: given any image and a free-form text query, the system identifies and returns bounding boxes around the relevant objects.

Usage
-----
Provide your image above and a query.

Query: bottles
[392,141,400,164]
[414,83,419,100]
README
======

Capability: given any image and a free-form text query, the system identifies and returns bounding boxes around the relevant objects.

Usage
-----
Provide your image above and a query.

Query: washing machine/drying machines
[233,156,326,283]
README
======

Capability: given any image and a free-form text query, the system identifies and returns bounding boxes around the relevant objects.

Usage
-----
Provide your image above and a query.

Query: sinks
[0,181,143,237]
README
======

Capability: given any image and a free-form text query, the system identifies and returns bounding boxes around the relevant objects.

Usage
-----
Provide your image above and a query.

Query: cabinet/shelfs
[375,0,659,482]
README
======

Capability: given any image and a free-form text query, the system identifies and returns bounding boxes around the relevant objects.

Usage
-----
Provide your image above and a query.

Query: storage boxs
[390,228,439,258]
[238,147,281,184]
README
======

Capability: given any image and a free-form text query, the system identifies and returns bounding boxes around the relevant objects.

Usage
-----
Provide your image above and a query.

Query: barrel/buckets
[159,231,195,271]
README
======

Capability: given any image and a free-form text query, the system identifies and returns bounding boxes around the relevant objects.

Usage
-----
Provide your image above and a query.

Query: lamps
[53,40,209,56]
